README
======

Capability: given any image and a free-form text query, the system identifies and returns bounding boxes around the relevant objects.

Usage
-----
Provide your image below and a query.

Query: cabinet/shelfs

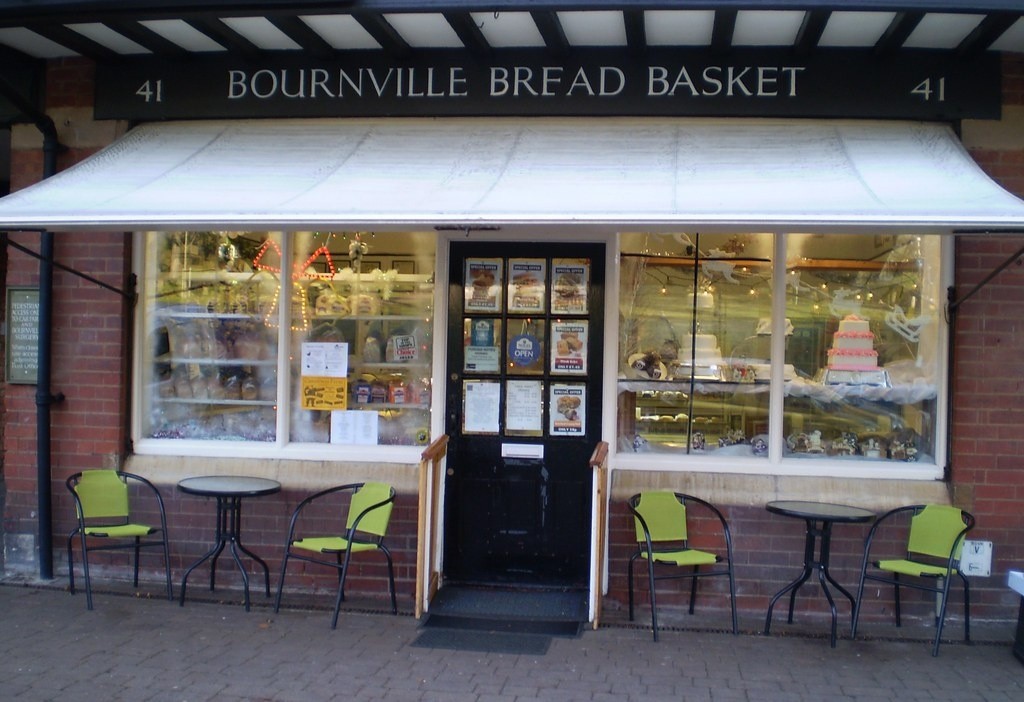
[152,270,433,411]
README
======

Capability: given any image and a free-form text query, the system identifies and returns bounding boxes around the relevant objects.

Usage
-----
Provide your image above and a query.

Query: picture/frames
[361,260,381,293]
[310,261,327,273]
[391,260,416,292]
[329,260,351,292]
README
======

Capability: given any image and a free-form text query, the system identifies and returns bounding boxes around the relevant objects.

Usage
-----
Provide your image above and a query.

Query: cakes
[756,318,794,334]
[746,363,794,381]
[827,314,879,371]
[678,333,722,364]
[686,292,713,308]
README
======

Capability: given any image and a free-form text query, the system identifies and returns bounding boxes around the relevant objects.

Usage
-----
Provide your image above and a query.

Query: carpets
[410,630,552,656]
[432,586,586,623]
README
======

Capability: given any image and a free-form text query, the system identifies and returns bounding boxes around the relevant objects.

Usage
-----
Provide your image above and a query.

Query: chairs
[626,490,738,643]
[273,483,398,631]
[849,504,975,657]
[65,468,173,611]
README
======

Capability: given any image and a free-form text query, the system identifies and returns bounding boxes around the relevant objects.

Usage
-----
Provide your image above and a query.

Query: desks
[175,475,281,613]
[765,500,877,649]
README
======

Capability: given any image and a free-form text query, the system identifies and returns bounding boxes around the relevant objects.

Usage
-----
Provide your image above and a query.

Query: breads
[159,334,278,401]
